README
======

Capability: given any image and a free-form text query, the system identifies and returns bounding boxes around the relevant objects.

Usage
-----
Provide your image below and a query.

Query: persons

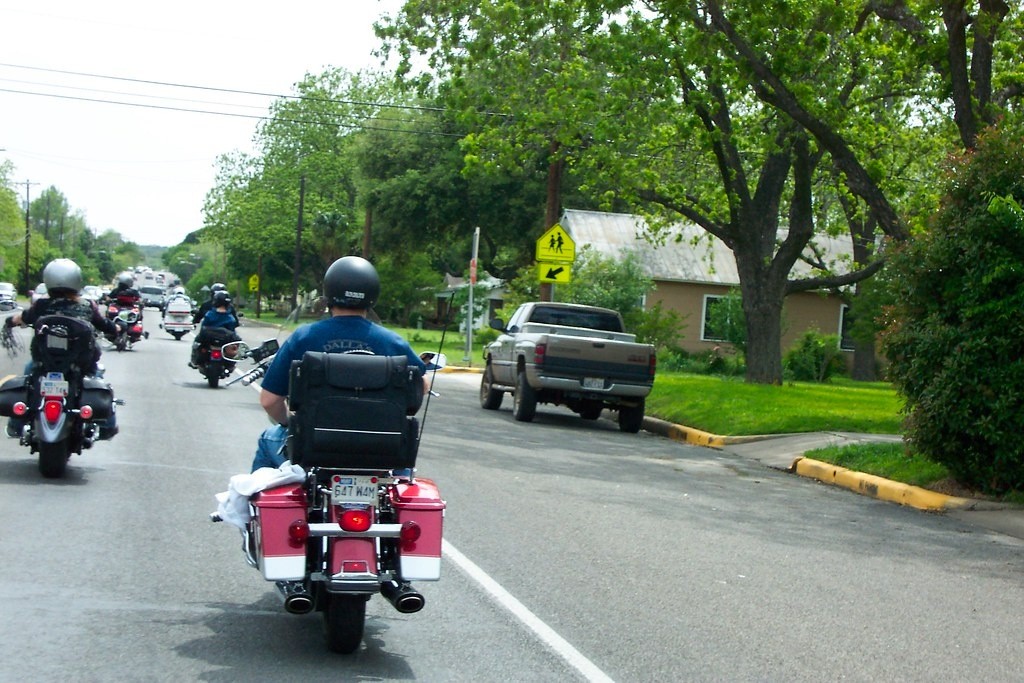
[5,259,242,437]
[251,256,431,476]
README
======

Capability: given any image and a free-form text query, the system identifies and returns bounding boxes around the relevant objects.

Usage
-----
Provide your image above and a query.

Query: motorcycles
[209,351,451,652]
[103,300,150,350]
[9,320,125,478]
[158,297,195,340]
[188,312,245,389]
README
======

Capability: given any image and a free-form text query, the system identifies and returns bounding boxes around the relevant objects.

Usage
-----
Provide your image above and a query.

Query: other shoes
[7,421,24,437]
[97,426,118,440]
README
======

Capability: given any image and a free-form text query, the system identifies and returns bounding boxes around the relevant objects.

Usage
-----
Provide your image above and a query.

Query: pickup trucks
[478,301,657,433]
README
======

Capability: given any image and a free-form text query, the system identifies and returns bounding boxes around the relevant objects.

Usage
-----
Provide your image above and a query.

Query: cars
[0,282,21,309]
[84,264,183,310]
[29,282,50,304]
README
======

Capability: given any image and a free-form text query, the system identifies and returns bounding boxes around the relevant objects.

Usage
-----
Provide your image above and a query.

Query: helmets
[322,256,380,310]
[43,258,81,295]
[174,286,185,294]
[210,283,226,298]
[118,274,133,288]
[212,291,231,305]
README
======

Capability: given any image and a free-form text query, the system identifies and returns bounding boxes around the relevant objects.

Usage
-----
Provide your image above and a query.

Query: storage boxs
[250,477,446,582]
